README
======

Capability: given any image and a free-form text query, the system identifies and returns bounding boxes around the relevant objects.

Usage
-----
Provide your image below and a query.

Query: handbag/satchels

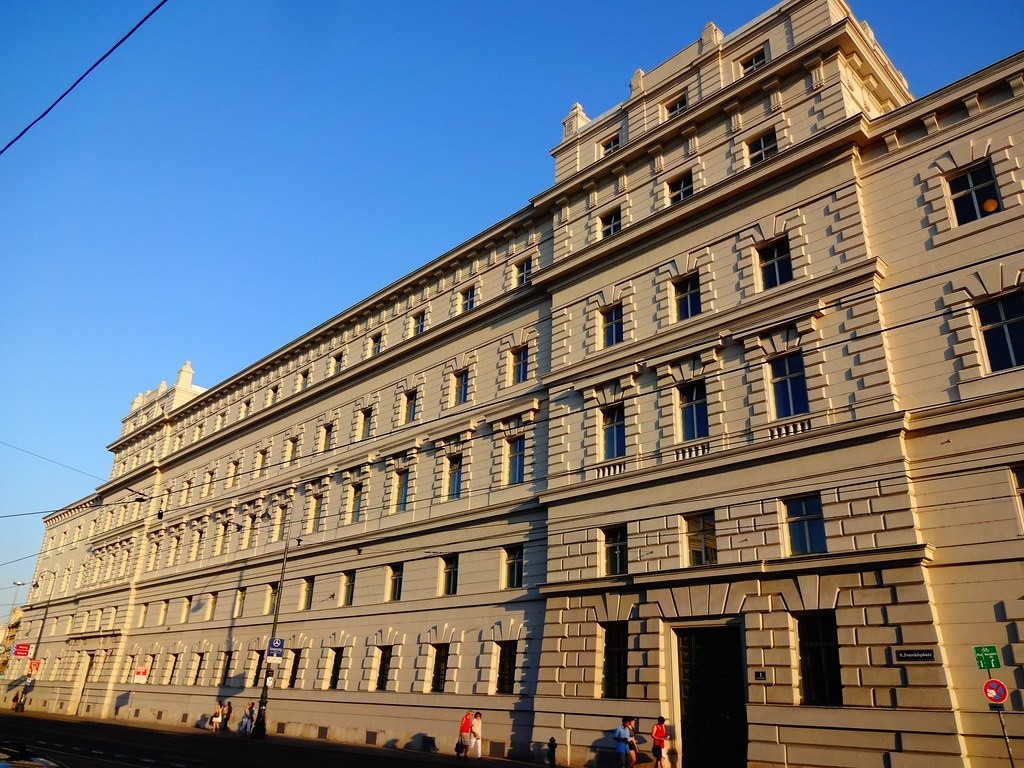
[455,738,463,753]
[215,711,219,717]
[629,741,635,750]
[222,707,227,714]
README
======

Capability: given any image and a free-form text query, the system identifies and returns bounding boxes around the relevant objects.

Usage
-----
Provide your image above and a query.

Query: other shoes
[478,758,483,760]
[464,757,469,761]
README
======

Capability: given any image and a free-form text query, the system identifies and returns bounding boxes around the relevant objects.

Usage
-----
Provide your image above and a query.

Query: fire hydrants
[548,737,557,768]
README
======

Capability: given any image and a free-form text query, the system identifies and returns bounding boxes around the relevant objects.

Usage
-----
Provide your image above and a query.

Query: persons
[613,717,640,768]
[466,711,482,759]
[651,716,670,768]
[238,701,257,733]
[212,700,233,734]
[12,690,27,712]
[456,710,478,757]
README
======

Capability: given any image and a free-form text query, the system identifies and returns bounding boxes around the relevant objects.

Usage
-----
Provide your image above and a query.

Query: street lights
[16,570,57,713]
[250,492,294,739]
